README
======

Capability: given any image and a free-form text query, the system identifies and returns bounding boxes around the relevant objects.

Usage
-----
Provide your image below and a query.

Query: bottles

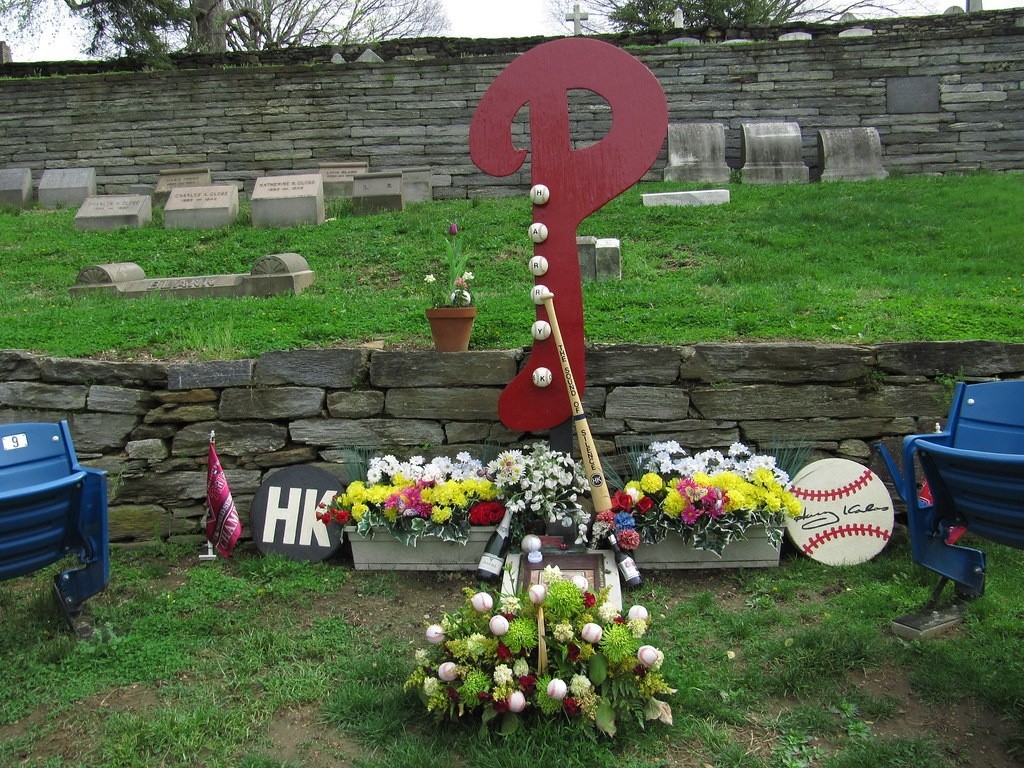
[607,529,644,589]
[477,509,513,583]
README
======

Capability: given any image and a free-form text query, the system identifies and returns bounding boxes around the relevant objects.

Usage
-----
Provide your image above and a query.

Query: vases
[345,522,786,569]
[425,308,477,351]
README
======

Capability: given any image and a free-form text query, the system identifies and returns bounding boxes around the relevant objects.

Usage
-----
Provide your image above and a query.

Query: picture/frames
[499,547,621,618]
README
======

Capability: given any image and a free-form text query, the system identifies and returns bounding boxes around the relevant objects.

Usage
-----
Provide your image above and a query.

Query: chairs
[874,378,1024,642]
[0,420,109,639]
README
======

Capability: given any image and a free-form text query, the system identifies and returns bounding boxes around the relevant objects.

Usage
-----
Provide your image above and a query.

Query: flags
[201,430,242,560]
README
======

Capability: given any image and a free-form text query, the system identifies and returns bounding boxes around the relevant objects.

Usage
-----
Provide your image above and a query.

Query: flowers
[428,222,478,308]
[401,536,684,732]
[320,435,809,565]
